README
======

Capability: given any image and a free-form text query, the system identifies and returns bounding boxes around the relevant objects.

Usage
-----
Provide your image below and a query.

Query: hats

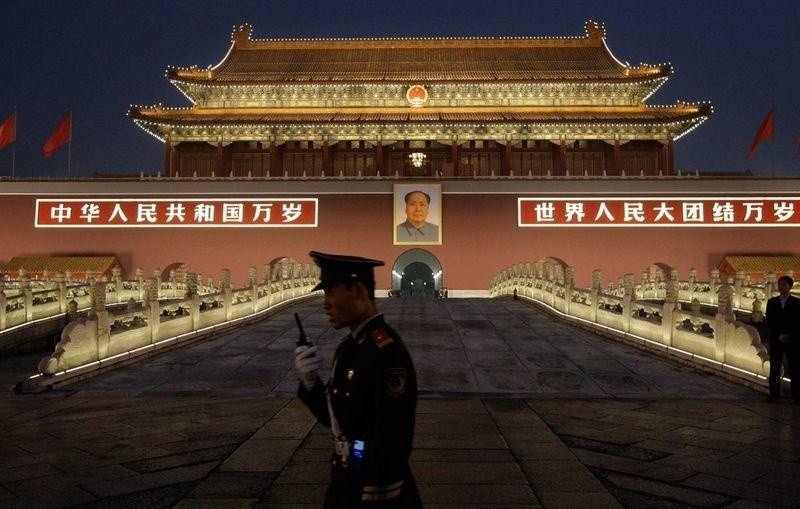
[310,250,386,292]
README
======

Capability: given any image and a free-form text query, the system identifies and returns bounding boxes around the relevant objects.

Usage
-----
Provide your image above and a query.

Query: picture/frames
[391,182,443,247]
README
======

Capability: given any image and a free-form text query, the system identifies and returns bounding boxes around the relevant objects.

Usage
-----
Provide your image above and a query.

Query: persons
[765,275,800,405]
[293,249,425,509]
[388,287,393,297]
[438,288,444,298]
[444,287,448,298]
[396,190,439,243]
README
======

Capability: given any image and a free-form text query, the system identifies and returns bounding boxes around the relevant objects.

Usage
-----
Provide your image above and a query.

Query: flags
[745,110,773,159]
[0,112,17,149]
[41,114,70,160]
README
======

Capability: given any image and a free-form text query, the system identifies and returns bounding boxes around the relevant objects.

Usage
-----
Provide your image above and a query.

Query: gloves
[294,343,323,390]
